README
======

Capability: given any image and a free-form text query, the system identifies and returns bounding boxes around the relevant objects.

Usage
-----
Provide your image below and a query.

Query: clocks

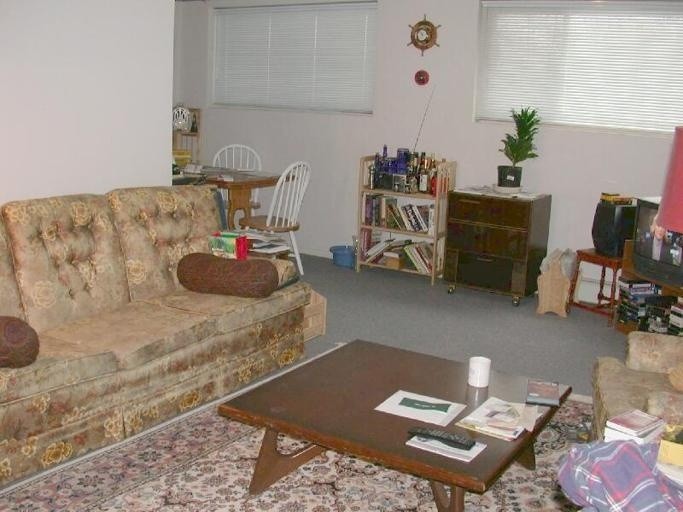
[406,14,442,58]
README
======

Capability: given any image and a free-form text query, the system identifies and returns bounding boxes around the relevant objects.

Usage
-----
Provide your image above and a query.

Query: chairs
[237,160,313,275]
[209,144,261,219]
[592,330,683,446]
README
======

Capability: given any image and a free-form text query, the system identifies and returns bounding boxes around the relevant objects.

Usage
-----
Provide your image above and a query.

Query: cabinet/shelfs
[615,239,683,333]
[354,155,457,286]
[443,187,552,298]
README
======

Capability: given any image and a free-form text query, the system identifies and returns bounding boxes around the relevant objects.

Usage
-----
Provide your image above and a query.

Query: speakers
[592,202,636,258]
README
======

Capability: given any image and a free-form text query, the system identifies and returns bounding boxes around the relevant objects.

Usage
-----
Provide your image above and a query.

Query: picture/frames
[188,108,204,136]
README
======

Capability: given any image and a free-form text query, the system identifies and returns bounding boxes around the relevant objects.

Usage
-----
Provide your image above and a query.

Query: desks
[566,247,620,330]
[172,161,301,233]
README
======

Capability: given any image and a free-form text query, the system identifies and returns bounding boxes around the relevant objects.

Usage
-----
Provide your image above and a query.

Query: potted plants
[495,104,540,190]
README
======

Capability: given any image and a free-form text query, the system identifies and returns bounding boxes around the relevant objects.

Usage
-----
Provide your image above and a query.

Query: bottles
[410,151,447,194]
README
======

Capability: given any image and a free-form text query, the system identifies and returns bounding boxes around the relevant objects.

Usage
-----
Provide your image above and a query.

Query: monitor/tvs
[633,198,683,284]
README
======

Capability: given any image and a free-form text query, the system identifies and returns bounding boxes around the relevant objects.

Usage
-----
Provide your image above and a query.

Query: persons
[636,210,673,264]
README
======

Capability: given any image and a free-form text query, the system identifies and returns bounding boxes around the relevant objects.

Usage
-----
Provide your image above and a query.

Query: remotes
[407,425,476,450]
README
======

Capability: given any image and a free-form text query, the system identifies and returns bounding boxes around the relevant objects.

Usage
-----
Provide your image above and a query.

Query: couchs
[0,184,313,492]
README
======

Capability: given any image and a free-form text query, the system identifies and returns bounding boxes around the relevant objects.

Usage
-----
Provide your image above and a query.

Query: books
[603,408,683,484]
[405,378,561,463]
[230,228,291,255]
[215,174,234,183]
[615,276,683,341]
[182,162,203,175]
[358,190,444,276]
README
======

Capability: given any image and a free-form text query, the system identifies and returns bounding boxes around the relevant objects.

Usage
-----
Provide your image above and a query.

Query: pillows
[174,252,281,298]
[0,316,40,370]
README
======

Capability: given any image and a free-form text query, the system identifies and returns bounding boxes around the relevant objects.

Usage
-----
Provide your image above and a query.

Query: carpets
[0,343,593,512]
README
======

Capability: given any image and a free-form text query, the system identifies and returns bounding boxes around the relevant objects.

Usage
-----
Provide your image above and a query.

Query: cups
[464,387,488,414]
[467,355,491,387]
